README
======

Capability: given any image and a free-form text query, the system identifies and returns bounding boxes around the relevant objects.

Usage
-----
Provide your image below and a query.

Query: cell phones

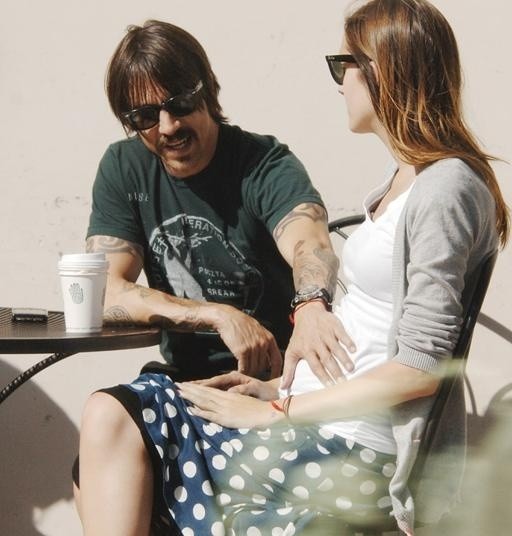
[12,304,49,322]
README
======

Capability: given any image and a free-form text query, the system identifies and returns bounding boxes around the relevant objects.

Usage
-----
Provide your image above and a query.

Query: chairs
[328,214,366,242]
[309,249,498,536]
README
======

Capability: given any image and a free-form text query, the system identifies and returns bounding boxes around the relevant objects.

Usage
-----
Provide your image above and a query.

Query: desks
[0,306,162,401]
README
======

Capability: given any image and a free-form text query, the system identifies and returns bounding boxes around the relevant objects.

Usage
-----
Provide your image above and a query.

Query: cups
[57,251,108,334]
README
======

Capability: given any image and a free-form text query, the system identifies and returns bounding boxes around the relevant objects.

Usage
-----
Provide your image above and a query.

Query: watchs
[290,285,332,309]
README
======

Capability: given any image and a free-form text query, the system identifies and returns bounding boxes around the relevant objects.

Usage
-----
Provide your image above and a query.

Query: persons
[83,18,358,393]
[71,0,512,536]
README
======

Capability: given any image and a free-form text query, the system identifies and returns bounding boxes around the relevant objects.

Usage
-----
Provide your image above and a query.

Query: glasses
[326,55,356,85]
[120,77,203,130]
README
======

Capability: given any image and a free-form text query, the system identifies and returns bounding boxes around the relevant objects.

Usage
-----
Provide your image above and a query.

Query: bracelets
[270,394,294,423]
[288,298,332,324]
